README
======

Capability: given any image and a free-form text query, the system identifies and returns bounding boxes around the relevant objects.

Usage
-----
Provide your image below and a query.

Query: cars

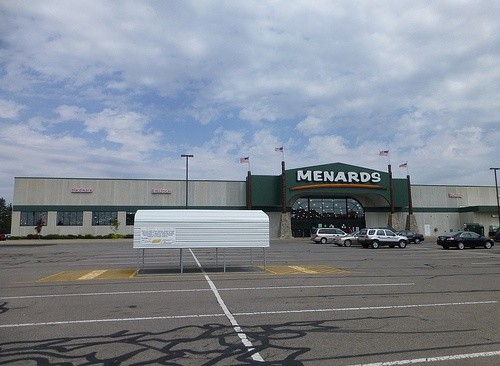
[394,230,424,244]
[335,231,361,247]
[437,231,495,250]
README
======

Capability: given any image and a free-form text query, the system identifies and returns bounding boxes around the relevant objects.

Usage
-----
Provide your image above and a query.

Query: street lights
[181,154,193,208]
[490,167,500,224]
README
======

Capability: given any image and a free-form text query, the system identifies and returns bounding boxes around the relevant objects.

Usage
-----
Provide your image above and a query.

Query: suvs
[359,229,409,248]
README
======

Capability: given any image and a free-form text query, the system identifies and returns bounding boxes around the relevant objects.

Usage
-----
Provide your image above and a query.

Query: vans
[310,228,348,243]
